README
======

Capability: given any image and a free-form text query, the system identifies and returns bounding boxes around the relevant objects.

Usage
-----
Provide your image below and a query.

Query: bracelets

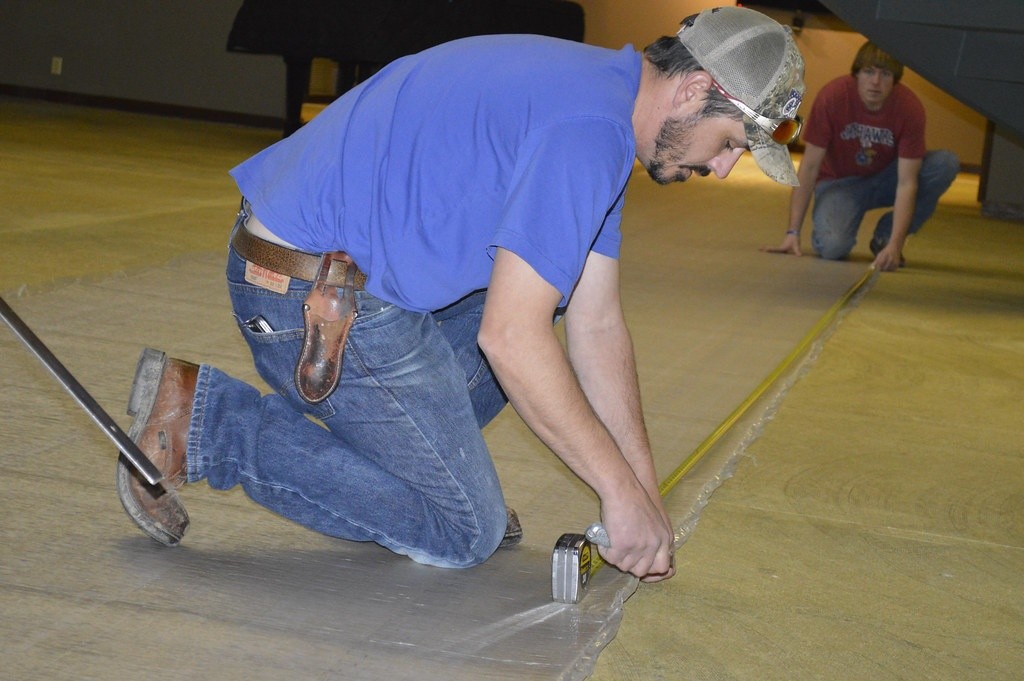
[787,230,800,235]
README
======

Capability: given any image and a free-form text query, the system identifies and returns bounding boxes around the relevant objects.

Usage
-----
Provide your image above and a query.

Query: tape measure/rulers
[552,258,885,602]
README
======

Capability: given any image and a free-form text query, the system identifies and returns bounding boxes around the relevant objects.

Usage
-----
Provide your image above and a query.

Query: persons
[766,41,958,273]
[116,9,803,583]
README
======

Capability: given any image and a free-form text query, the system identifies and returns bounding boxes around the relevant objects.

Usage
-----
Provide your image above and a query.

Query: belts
[229,222,367,291]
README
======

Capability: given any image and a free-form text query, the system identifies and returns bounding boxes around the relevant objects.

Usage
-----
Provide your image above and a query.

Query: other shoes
[869,235,906,268]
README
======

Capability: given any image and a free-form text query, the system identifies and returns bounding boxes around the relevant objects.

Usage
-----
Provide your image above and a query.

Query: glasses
[712,81,805,145]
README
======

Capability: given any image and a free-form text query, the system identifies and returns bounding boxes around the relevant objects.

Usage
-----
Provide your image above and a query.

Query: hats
[677,6,806,187]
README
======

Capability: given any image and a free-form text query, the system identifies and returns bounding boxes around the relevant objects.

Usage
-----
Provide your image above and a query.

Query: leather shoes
[118,347,200,546]
[496,505,523,548]
